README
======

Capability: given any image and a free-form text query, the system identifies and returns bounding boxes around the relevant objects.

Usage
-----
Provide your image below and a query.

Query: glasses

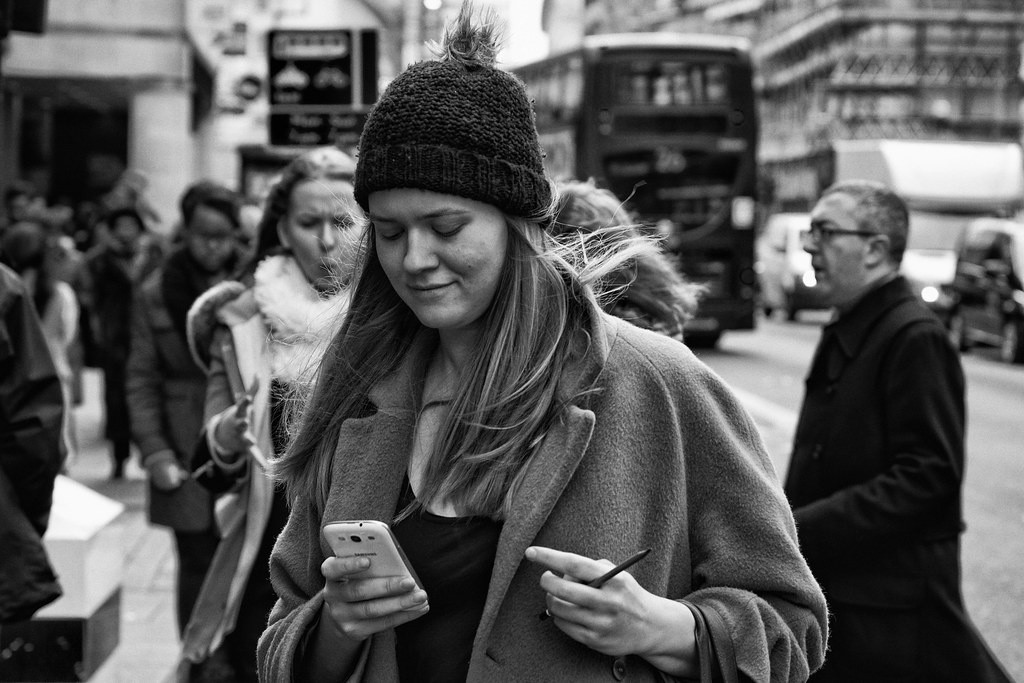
[799,227,882,245]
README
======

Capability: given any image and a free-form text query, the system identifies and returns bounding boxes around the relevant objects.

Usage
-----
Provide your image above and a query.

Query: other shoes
[108,464,128,481]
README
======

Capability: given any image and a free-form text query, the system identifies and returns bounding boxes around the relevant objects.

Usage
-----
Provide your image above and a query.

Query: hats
[353,0,554,221]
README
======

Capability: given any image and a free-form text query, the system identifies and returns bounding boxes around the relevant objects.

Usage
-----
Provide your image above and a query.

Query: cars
[931,219,1023,366]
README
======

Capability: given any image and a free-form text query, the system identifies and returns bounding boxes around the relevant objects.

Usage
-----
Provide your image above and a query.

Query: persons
[783,178,1014,683]
[255,21,829,683]
[0,163,179,641]
[126,178,255,641]
[165,145,370,683]
[550,175,708,342]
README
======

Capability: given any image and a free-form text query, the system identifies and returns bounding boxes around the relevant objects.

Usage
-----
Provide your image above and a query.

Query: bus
[506,32,777,351]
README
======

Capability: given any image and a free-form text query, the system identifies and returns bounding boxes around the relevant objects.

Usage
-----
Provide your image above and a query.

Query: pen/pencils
[537,546,652,621]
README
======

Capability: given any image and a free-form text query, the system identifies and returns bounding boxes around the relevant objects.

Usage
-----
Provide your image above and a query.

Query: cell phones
[322,520,429,611]
